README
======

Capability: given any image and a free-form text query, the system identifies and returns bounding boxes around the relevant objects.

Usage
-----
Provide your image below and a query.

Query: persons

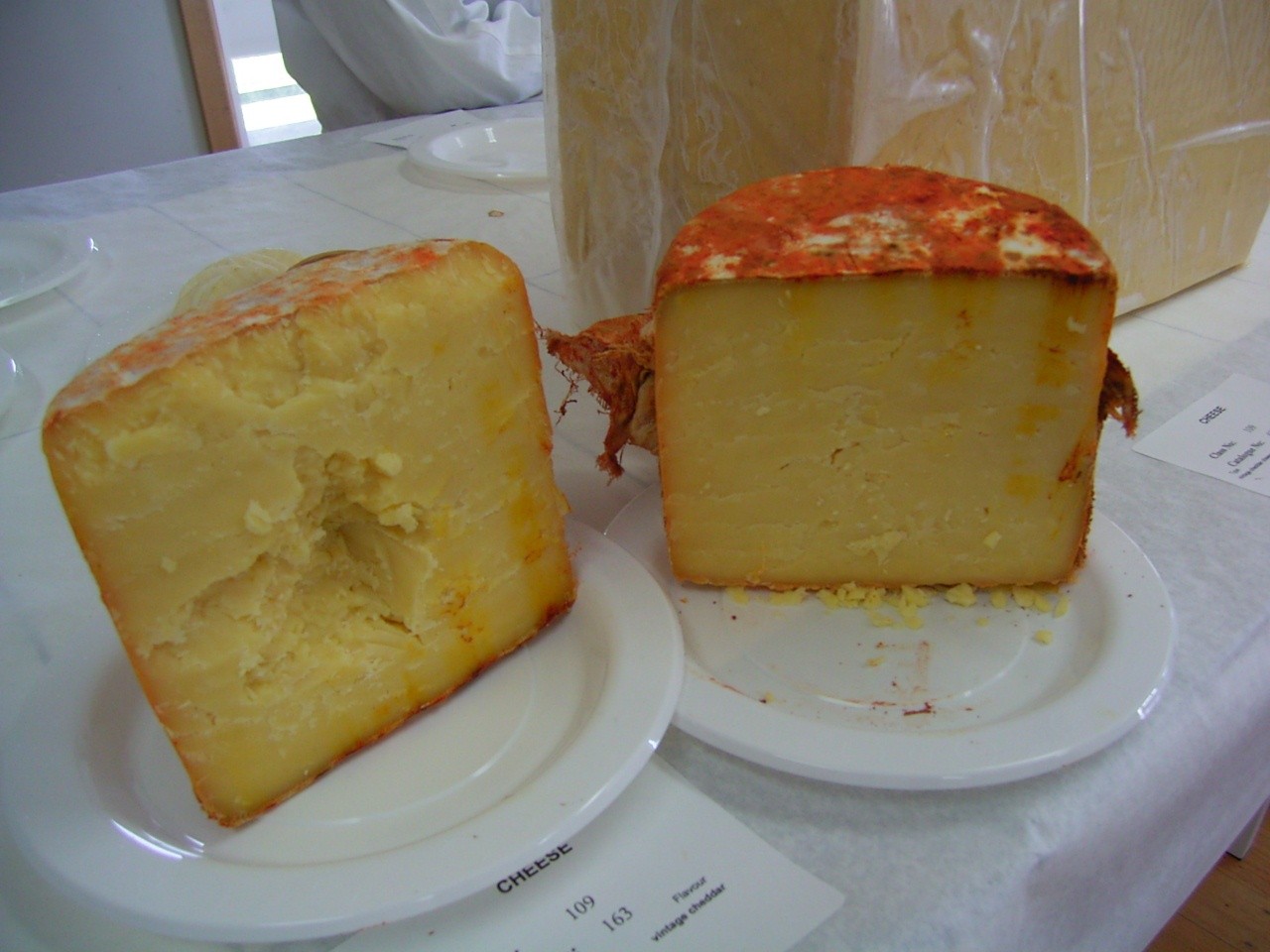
[271,0,541,136]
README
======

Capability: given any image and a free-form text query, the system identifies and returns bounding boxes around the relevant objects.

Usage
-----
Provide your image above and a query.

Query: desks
[0,91,1270,952]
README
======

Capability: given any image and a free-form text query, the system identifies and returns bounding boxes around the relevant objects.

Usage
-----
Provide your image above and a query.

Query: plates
[406,117,548,179]
[601,479,1177,791]
[0,516,685,944]
[0,219,94,308]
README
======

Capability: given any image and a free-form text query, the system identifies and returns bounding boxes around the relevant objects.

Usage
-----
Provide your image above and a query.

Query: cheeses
[37,231,574,831]
[645,162,1120,596]
